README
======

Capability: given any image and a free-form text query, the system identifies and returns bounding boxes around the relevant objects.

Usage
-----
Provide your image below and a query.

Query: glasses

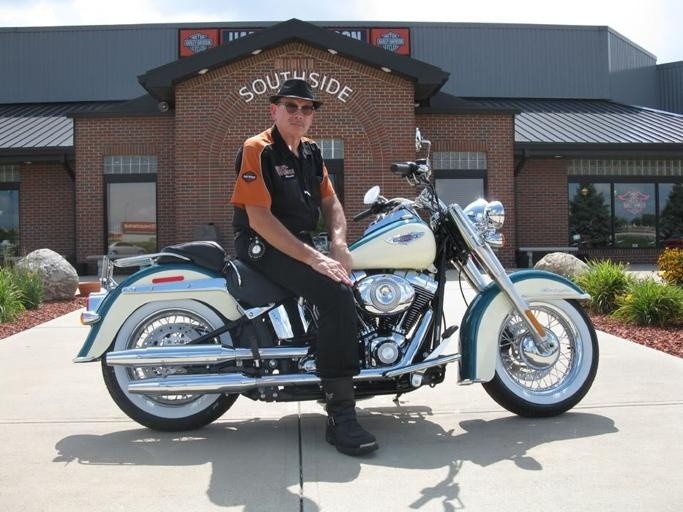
[274,101,315,117]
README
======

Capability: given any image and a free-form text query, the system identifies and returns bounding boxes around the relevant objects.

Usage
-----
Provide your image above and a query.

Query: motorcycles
[71,126,599,433]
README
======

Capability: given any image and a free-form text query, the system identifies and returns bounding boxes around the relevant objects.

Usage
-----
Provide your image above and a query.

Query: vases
[78,282,100,297]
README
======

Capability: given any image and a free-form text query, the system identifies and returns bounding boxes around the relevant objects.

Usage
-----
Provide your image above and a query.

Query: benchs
[518,246,578,268]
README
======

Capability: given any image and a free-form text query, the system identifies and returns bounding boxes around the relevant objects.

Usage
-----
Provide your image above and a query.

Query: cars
[108,241,145,256]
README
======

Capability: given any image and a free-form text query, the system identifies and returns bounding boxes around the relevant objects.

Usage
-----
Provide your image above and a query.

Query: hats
[268,78,324,110]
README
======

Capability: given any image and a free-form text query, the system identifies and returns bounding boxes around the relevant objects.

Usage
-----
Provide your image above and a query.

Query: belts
[230,229,313,238]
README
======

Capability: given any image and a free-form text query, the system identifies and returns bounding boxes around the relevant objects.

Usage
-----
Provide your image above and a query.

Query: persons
[227,76,380,456]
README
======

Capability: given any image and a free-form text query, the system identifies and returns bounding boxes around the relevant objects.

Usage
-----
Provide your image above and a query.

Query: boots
[320,375,381,457]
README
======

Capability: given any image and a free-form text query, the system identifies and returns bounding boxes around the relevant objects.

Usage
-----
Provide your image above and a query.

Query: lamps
[158,102,169,113]
